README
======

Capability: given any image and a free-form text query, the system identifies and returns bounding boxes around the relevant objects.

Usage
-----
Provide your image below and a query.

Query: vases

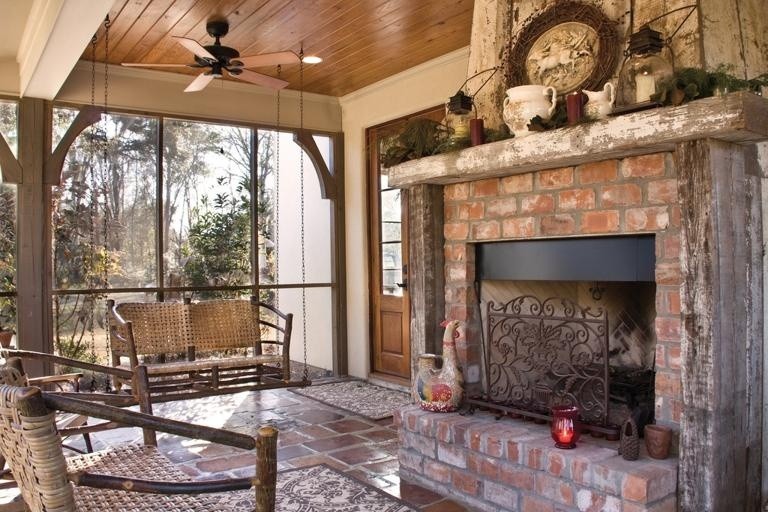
[501,85,556,137]
[549,404,582,450]
[412,319,465,413]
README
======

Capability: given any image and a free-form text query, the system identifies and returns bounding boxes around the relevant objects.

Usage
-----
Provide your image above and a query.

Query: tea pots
[580,82,614,121]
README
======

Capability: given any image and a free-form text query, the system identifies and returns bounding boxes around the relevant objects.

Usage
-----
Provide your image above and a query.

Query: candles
[566,90,583,126]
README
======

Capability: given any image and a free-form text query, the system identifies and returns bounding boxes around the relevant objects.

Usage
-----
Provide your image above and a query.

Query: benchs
[106,295,294,397]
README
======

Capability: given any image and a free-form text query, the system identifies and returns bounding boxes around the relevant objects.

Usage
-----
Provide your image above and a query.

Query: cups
[551,405,581,450]
[565,92,585,127]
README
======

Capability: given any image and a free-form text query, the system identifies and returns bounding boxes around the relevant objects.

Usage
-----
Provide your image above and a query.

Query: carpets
[288,378,410,421]
[207,463,418,512]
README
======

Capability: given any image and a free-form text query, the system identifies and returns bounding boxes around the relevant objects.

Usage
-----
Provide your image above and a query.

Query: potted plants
[0,303,17,347]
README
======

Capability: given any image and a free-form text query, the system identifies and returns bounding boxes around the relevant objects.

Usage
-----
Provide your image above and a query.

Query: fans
[121,18,301,93]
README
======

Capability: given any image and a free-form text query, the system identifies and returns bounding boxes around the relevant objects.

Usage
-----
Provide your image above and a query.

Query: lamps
[615,4,696,113]
[436,67,498,147]
